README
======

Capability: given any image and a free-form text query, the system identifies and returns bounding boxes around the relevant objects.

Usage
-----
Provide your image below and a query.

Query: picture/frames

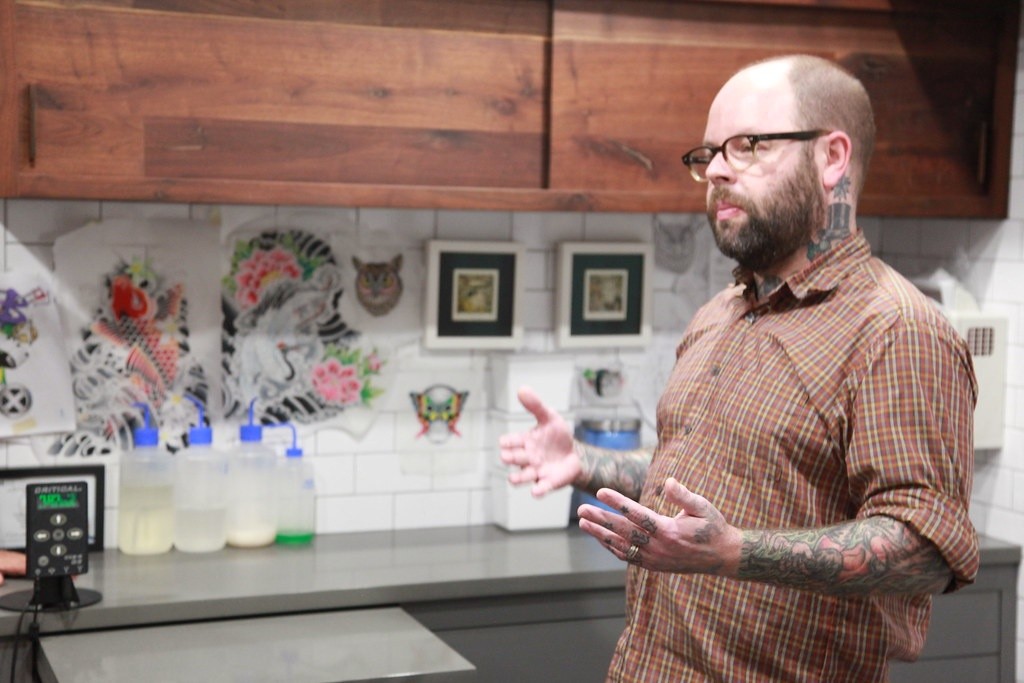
[556,240,655,349]
[425,238,525,351]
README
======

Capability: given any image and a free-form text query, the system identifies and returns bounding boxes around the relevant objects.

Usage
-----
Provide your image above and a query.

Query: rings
[626,543,639,559]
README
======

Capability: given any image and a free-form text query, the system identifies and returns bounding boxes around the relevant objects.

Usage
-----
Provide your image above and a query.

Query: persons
[500,49,979,683]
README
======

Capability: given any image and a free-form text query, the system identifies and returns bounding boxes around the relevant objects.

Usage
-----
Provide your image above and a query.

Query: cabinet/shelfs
[1,564,1024,682]
[1,1,1024,221]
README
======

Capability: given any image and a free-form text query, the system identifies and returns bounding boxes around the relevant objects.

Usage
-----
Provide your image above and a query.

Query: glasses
[680,128,832,183]
